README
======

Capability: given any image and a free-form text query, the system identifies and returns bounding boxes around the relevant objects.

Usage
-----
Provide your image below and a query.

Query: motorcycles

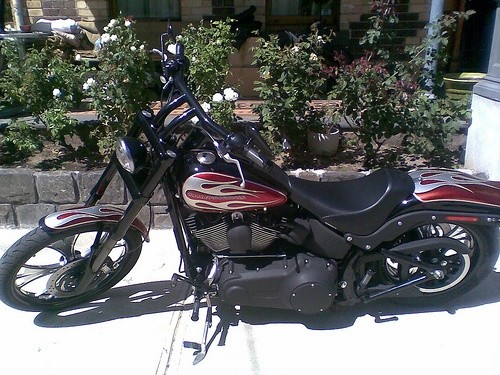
[1,19,500,365]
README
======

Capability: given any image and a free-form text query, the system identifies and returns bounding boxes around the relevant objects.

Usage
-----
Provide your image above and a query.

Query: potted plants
[307,101,340,157]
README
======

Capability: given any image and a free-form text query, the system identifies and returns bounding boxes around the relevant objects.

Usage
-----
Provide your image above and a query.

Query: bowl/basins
[20,25,31,31]
